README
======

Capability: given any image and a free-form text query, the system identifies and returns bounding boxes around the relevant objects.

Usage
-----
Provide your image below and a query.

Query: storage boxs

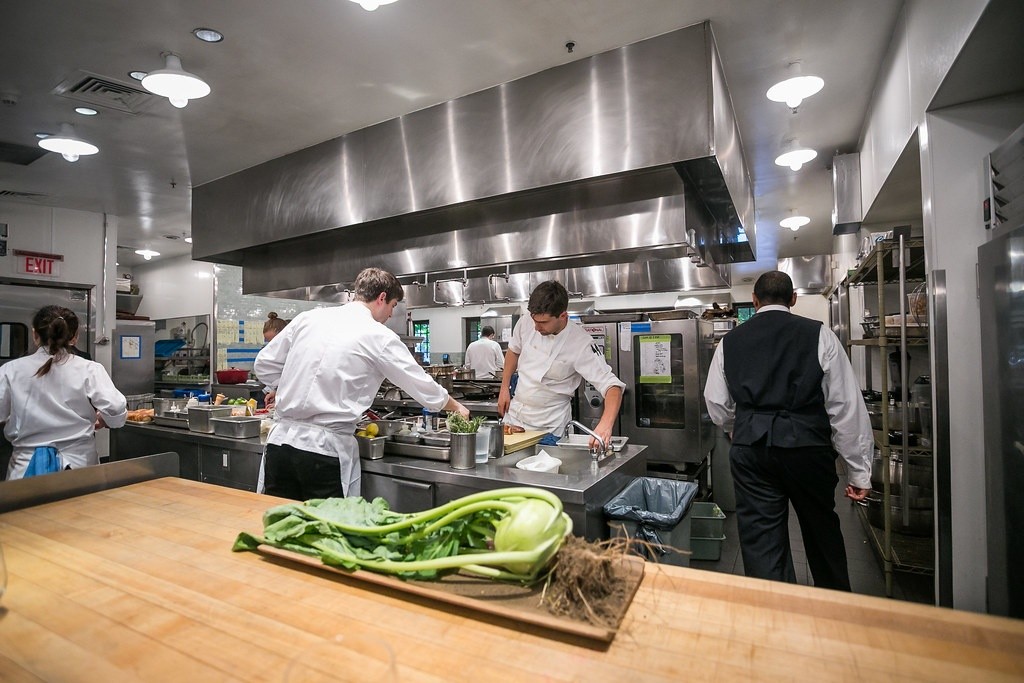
[116,278,131,292]
[689,502,726,560]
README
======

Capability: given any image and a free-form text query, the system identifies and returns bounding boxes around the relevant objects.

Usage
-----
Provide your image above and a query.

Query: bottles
[399,417,425,437]
[198,394,211,405]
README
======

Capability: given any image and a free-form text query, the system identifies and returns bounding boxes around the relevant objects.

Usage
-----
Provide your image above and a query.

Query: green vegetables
[230,485,573,582]
[445,410,488,434]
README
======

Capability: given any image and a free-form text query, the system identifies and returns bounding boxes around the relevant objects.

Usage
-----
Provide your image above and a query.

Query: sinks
[463,394,499,402]
[484,444,617,476]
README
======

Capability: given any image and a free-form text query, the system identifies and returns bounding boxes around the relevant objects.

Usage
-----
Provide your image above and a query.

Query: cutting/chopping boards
[504,429,550,455]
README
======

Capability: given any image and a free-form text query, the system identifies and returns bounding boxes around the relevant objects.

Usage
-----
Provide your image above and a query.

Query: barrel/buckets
[907,292,926,314]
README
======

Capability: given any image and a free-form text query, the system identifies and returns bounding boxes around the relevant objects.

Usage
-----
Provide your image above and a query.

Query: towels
[538,432,561,446]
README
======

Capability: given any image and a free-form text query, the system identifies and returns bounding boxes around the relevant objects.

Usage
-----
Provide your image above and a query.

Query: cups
[449,432,477,470]
[475,426,491,464]
[481,421,505,459]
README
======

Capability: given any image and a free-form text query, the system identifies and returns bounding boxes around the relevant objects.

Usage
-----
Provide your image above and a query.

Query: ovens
[577,317,716,464]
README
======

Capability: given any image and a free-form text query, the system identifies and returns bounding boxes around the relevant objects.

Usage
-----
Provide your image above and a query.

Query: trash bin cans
[603,477,699,567]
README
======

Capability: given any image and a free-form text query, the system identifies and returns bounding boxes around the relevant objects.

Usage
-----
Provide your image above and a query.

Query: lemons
[356,423,378,439]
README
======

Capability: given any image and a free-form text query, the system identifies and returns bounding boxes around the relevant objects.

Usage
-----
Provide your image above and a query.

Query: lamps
[766,62,824,107]
[140,51,211,108]
[774,140,817,172]
[37,123,99,162]
[779,209,810,231]
[135,245,160,260]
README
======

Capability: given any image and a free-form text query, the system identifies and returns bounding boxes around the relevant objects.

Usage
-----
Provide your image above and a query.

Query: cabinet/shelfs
[844,234,935,595]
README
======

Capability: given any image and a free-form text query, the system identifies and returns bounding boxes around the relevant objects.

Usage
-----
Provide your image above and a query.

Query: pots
[861,379,934,535]
[213,367,251,384]
[424,364,518,394]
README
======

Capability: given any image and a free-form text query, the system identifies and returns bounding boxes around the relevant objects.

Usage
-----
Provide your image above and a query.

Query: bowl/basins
[885,313,926,324]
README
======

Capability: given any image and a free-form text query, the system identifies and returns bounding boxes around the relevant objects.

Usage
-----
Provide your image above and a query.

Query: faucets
[469,380,496,395]
[564,419,617,470]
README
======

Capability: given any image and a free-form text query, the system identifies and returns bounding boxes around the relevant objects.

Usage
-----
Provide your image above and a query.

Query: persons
[464,326,505,379]
[498,280,626,452]
[703,271,874,593]
[0,305,128,481]
[253,267,469,501]
[263,312,288,410]
